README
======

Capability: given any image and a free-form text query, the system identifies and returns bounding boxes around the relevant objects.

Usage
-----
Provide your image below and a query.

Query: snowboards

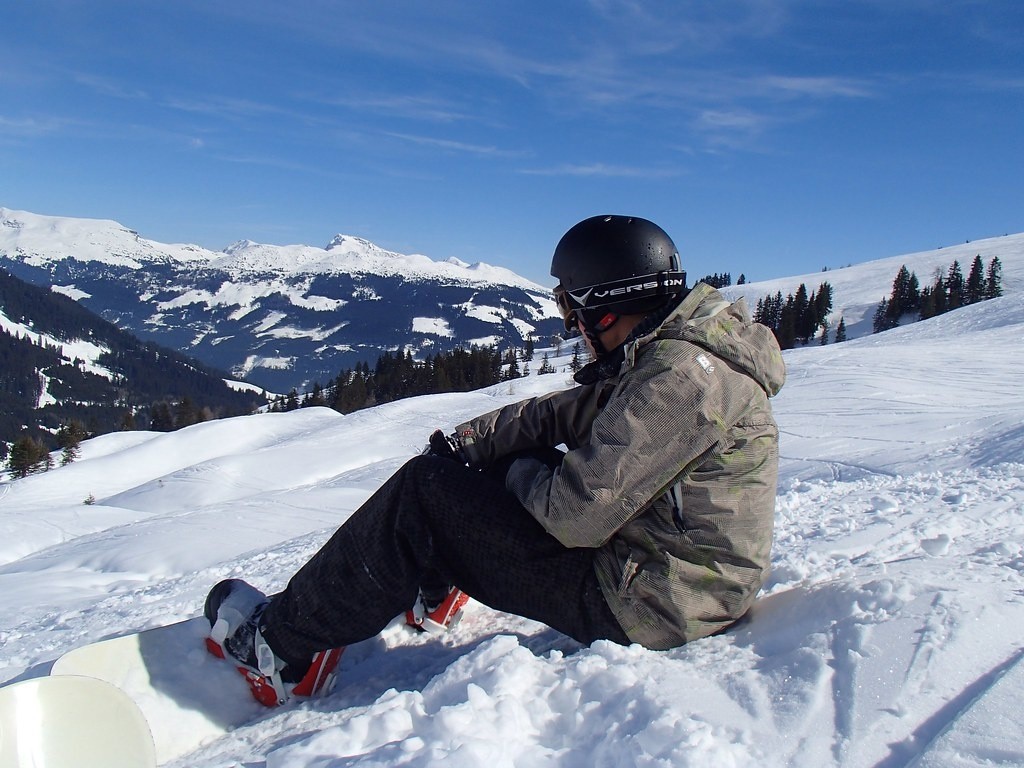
[0,589,489,768]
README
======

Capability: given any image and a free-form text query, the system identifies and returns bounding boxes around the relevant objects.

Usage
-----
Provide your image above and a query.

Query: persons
[202,211,786,708]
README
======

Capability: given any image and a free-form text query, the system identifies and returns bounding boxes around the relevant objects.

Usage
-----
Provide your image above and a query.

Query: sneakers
[204,578,313,686]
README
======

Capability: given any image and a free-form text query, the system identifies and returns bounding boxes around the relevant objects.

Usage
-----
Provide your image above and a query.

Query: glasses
[552,285,577,334]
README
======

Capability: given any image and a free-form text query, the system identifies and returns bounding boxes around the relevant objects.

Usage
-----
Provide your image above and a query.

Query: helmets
[550,215,683,328]
